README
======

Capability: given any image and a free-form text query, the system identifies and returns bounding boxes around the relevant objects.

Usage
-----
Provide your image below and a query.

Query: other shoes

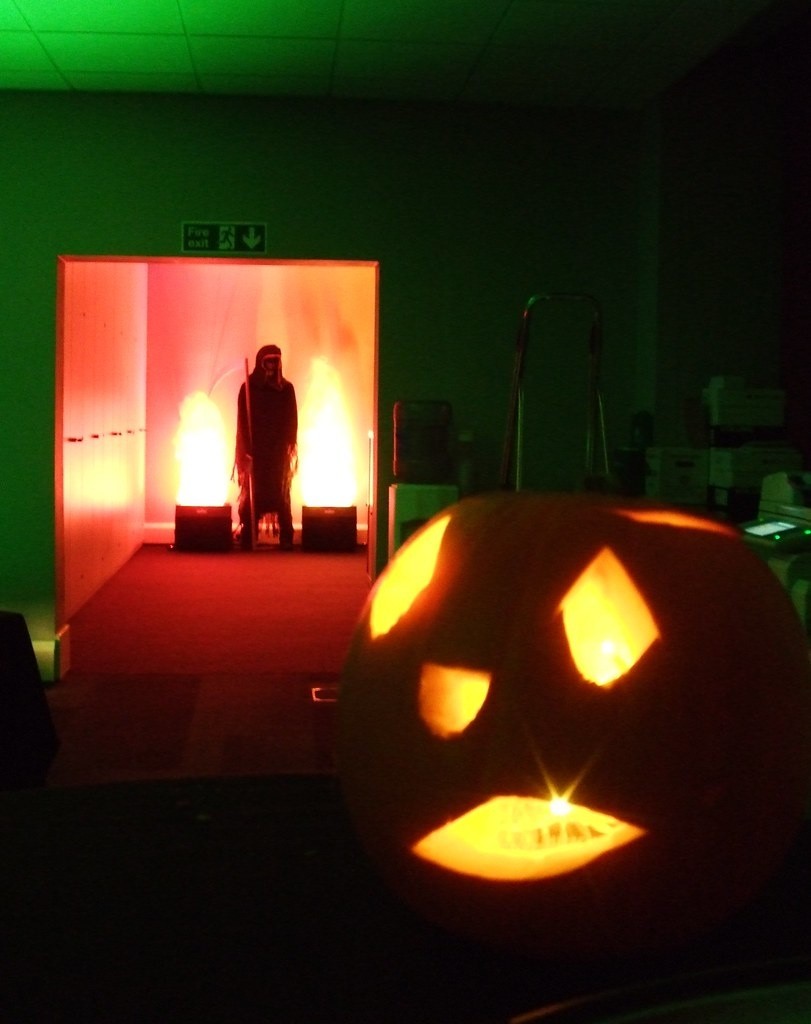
[280,543,292,551]
[240,543,252,551]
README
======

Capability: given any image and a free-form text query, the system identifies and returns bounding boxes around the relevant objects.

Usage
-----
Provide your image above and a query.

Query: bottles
[393,399,453,481]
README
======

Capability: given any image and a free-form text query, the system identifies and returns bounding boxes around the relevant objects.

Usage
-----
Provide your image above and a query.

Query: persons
[233,345,298,549]
